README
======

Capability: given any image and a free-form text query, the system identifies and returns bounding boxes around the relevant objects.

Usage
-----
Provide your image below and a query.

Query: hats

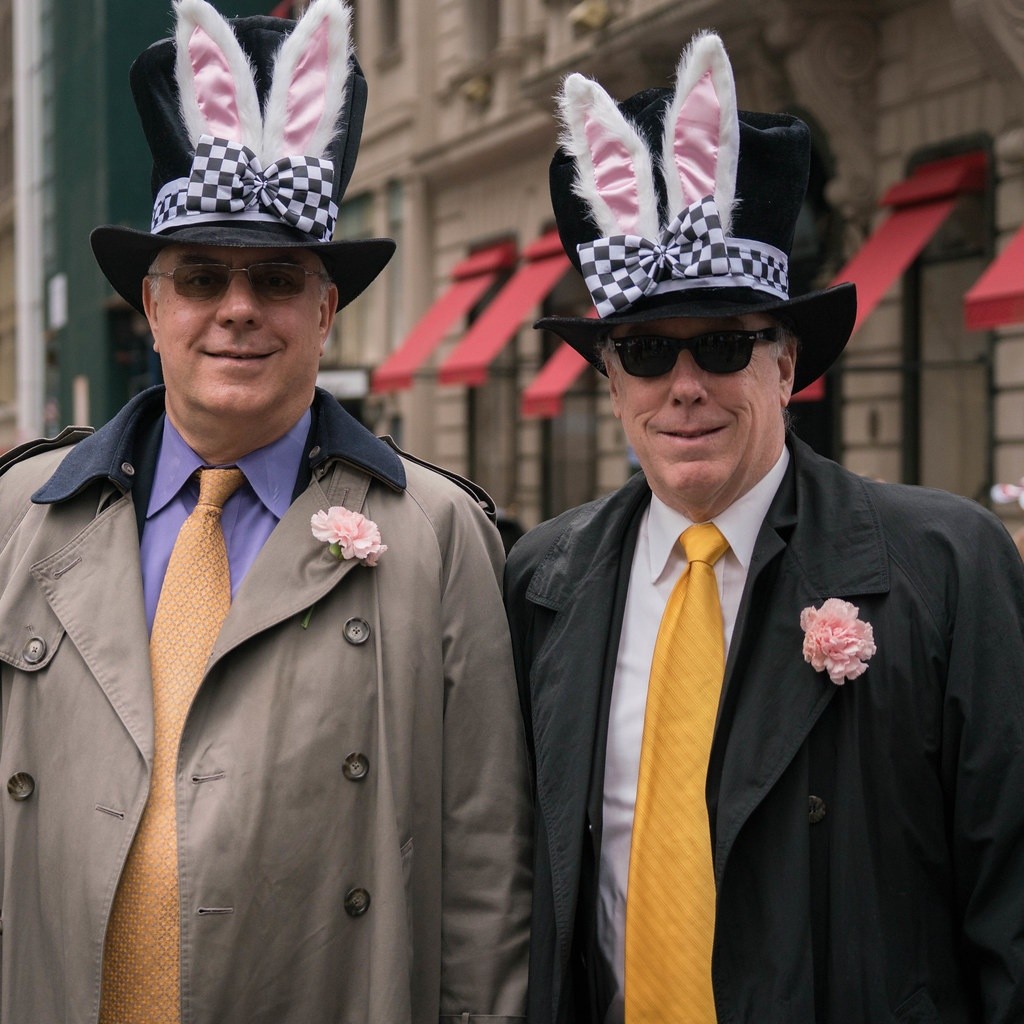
[89,0,396,318]
[531,27,858,396]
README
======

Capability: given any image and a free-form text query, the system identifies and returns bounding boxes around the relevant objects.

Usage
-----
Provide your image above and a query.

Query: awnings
[371,240,515,393]
[436,230,572,384]
[967,230,1024,331]
[521,304,602,418]
[788,155,989,396]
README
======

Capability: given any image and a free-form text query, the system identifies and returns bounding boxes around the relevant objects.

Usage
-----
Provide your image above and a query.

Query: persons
[504,30,1024,1024]
[1,0,531,1024]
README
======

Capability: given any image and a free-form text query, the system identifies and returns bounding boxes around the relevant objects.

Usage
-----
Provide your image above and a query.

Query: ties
[94,469,247,1024]
[623,523,728,1024]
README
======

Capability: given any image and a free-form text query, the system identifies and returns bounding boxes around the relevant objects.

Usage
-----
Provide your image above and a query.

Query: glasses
[147,261,328,302]
[605,322,778,379]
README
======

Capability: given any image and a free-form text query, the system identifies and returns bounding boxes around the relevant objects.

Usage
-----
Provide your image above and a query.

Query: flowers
[302,505,389,627]
[800,597,877,686]
